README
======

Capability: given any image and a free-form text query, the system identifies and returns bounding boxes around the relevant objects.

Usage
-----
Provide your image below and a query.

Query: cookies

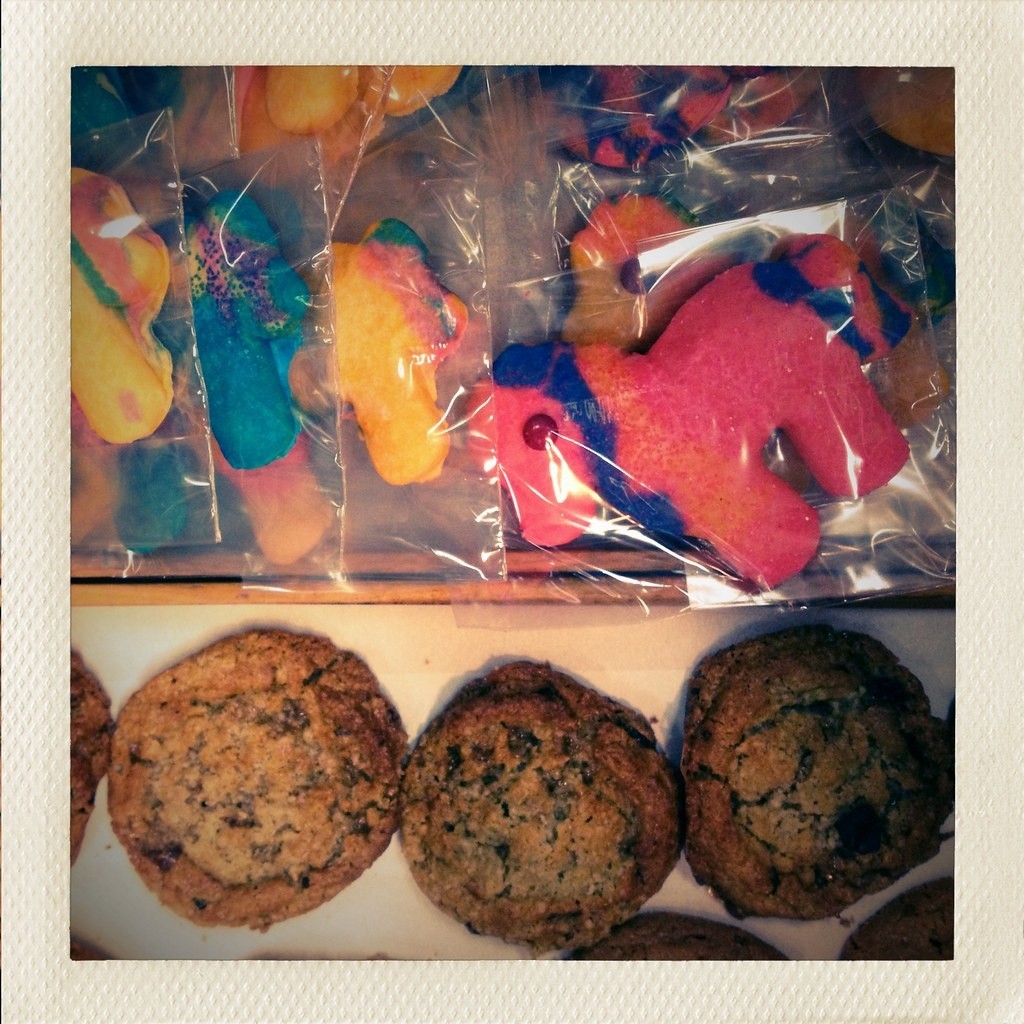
[71,66,951,591]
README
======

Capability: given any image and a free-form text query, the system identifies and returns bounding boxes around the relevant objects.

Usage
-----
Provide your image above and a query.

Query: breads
[70,621,956,963]
